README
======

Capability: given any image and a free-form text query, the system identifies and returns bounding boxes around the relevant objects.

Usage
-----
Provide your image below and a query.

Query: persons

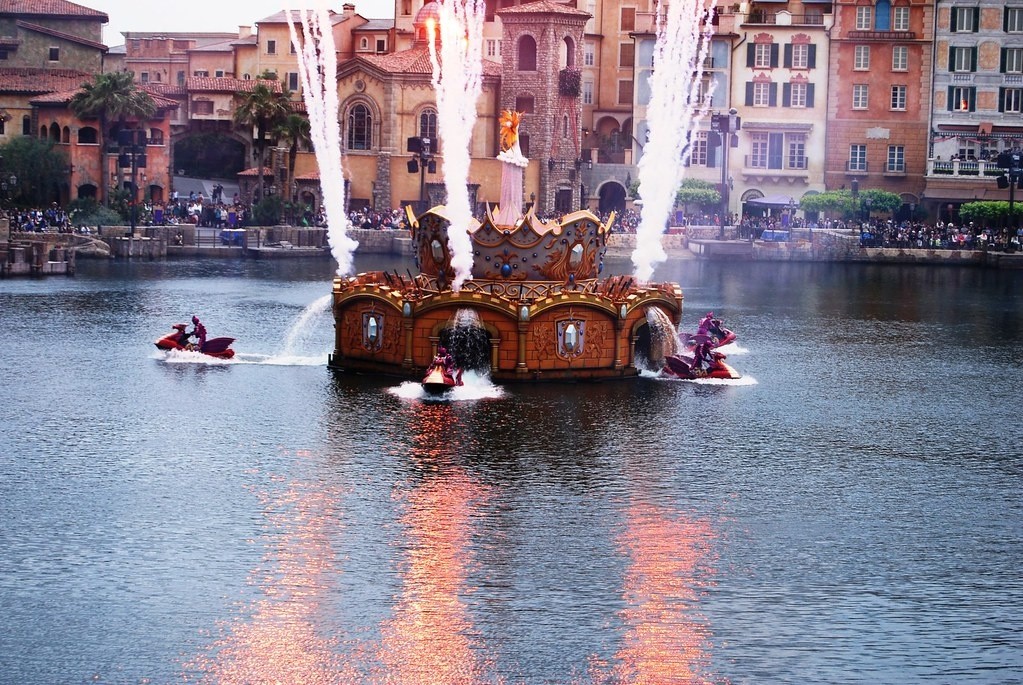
[711,7,718,25]
[932,143,1023,169]
[189,315,207,349]
[426,346,454,376]
[108,188,258,230]
[209,184,218,204]
[689,340,715,371]
[216,183,224,204]
[521,205,643,234]
[0,198,103,237]
[142,135,162,147]
[282,197,412,231]
[701,9,708,25]
[697,311,717,336]
[469,208,488,224]
[663,206,1023,253]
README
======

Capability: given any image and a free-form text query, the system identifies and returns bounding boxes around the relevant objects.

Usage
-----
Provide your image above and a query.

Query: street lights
[9,175,18,207]
[789,196,795,231]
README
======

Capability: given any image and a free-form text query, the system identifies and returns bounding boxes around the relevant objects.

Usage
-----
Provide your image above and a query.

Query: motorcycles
[661,349,741,381]
[419,346,465,395]
[667,320,736,350]
[154,322,238,359]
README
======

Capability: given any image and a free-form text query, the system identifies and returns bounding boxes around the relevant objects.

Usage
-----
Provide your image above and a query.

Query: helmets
[715,319,723,326]
[706,312,714,319]
[704,340,712,349]
[437,348,447,357]
[192,316,199,324]
